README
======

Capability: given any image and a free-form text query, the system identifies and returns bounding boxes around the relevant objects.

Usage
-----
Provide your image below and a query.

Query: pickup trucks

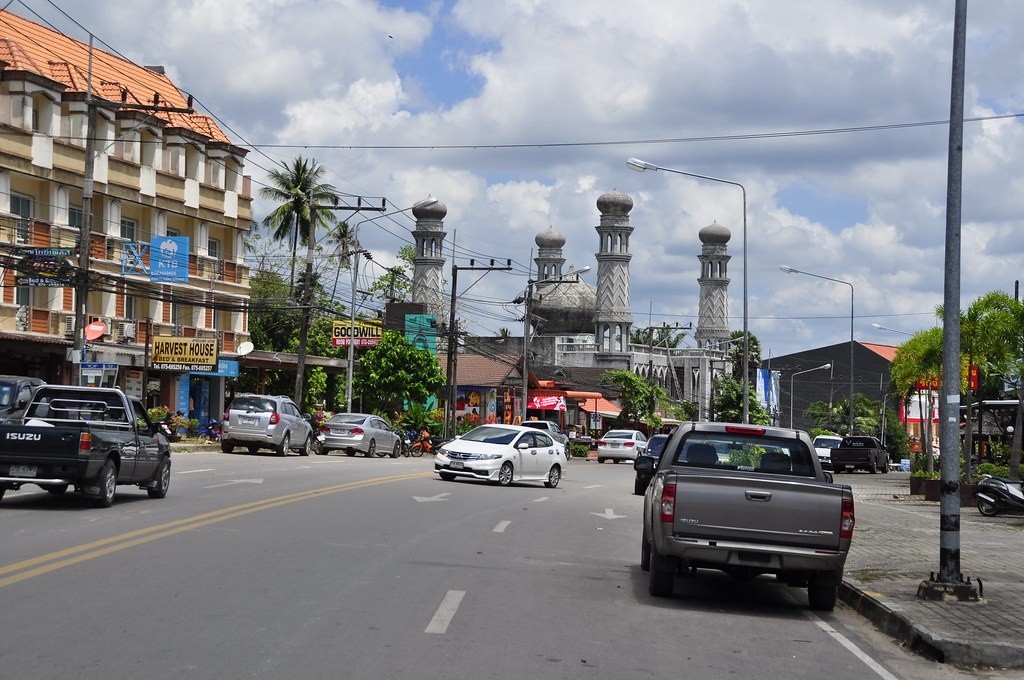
[633,422,856,612]
[830,435,890,474]
[0,384,173,509]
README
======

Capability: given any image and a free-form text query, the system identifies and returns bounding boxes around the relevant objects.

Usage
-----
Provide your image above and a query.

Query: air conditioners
[65,315,76,332]
[92,317,111,335]
[118,322,135,339]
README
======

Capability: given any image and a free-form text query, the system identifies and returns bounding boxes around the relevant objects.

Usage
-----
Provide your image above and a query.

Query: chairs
[760,452,791,472]
[686,444,718,465]
[46,404,69,418]
[109,406,123,421]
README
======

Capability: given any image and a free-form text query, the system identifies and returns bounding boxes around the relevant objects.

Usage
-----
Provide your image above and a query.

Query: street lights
[521,265,592,424]
[698,336,749,422]
[778,265,854,437]
[346,199,442,412]
[626,157,750,425]
[790,363,831,429]
[870,323,928,453]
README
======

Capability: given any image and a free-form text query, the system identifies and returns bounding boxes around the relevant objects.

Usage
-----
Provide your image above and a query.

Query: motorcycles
[400,428,455,458]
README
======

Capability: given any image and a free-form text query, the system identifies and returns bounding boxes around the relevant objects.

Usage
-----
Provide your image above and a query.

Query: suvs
[812,434,849,471]
[221,393,314,457]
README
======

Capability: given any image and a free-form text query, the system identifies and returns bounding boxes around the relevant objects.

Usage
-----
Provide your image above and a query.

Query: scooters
[291,429,323,455]
[196,416,221,444]
[975,472,1024,517]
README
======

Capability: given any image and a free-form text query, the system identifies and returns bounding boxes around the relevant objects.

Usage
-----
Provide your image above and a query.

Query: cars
[634,433,703,496]
[521,420,571,461]
[316,412,402,458]
[597,429,648,464]
[433,423,568,489]
[0,375,51,422]
[702,440,793,472]
